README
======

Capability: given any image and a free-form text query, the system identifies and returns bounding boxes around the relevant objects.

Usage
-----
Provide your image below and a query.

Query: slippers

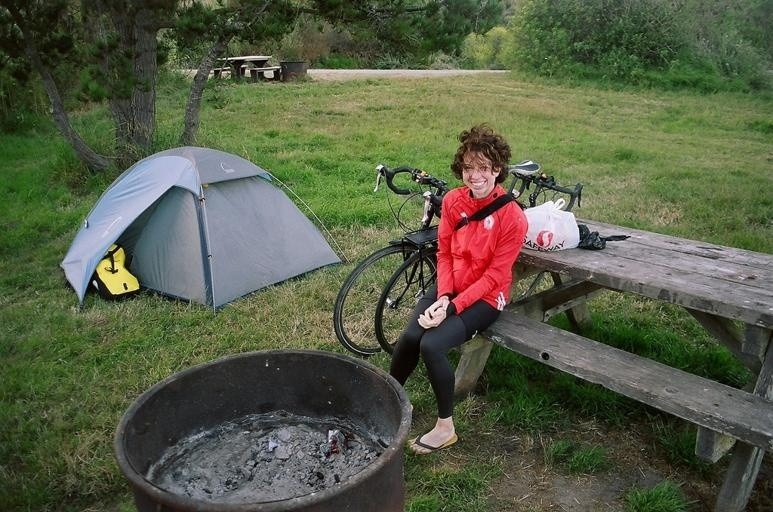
[409,434,459,455]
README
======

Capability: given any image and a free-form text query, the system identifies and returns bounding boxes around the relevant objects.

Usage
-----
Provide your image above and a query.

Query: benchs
[454,310,773,456]
[250,66,282,82]
[214,65,248,80]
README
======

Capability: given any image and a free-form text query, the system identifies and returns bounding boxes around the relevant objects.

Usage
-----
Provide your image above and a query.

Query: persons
[388,123,529,454]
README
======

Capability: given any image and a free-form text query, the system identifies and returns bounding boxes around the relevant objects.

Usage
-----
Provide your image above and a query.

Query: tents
[56,143,346,316]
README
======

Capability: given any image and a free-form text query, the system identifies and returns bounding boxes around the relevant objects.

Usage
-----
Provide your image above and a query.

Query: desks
[217,56,272,82]
[454,219,773,512]
